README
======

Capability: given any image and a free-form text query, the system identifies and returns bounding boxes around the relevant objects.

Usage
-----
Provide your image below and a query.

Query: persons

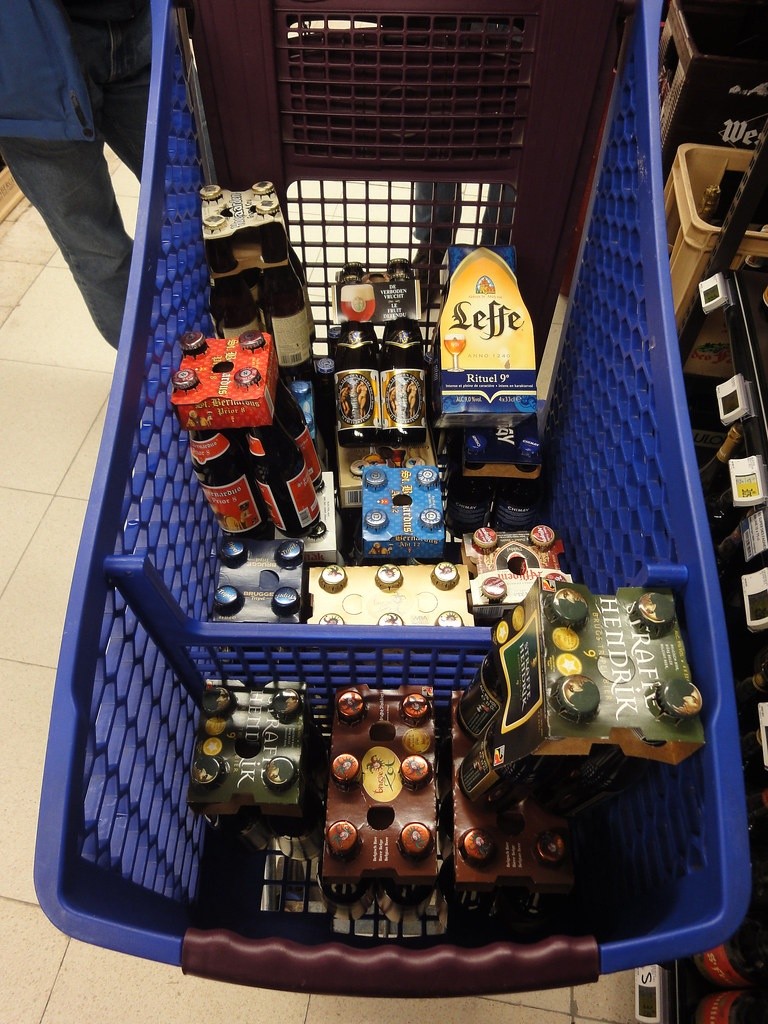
[0,1,155,356]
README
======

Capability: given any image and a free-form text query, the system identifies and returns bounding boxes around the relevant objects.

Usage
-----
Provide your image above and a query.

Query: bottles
[169,179,768,1024]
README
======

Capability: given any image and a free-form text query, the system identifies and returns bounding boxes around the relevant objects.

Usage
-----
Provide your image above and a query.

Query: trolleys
[35,2,753,996]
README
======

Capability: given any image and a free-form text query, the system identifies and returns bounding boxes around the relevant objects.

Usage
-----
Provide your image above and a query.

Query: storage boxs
[661,0,767,185]
[666,144,768,375]
